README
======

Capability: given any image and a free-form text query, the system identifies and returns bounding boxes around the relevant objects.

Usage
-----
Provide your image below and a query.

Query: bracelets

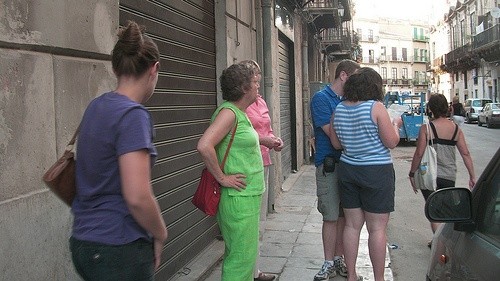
[409,172,415,177]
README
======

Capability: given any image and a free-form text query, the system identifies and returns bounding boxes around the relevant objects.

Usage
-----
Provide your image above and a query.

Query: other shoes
[254,273,275,281]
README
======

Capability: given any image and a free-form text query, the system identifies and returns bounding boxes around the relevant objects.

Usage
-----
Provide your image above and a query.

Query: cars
[478,102,500,128]
[422,146,500,281]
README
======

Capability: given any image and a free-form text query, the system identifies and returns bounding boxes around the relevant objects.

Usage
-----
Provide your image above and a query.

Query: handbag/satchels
[191,167,220,217]
[43,125,80,207]
[413,123,437,192]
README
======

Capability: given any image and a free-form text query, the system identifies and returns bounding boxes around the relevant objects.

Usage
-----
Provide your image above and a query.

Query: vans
[462,98,494,124]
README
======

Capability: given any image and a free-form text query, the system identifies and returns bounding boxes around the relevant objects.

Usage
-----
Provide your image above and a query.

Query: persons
[237,60,286,281]
[329,67,403,280]
[68,20,168,281]
[310,59,367,280]
[449,97,469,129]
[408,94,476,252]
[196,61,266,281]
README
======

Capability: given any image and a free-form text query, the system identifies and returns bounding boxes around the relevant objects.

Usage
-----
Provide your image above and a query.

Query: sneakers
[335,254,348,276]
[314,260,336,281]
